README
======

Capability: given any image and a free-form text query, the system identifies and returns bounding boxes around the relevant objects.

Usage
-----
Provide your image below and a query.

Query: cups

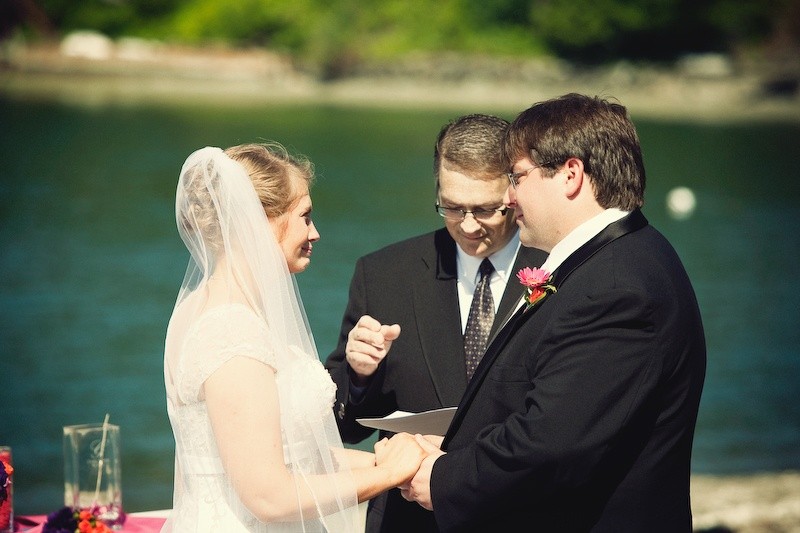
[61,423,123,530]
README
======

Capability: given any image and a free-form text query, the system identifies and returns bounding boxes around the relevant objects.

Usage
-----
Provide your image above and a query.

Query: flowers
[42,506,126,533]
[516,266,557,314]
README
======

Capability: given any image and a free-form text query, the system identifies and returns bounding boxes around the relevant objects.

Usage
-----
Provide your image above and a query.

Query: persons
[322,114,549,533]
[159,142,429,533]
[397,92,706,532]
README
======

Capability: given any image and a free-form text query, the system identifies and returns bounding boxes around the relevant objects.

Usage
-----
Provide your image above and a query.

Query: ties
[464,257,494,381]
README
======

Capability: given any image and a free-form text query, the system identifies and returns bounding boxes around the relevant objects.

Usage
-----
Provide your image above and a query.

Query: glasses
[507,162,549,190]
[435,181,508,221]
